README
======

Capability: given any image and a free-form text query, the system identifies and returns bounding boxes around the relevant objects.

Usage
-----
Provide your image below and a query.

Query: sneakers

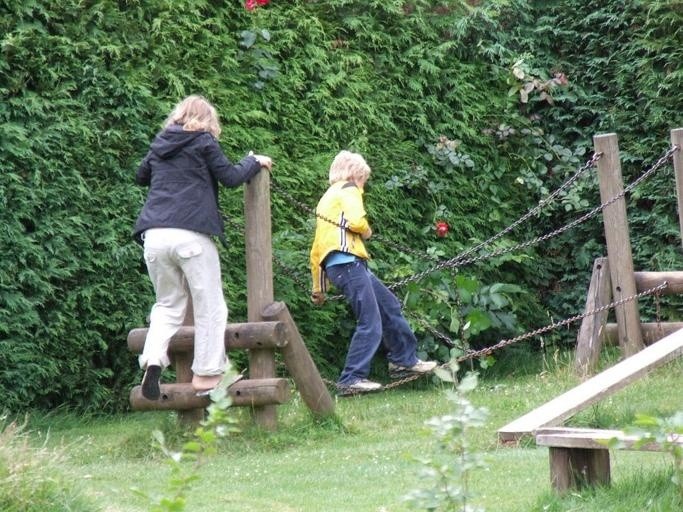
[388,358,438,376]
[335,377,381,398]
[139,360,163,403]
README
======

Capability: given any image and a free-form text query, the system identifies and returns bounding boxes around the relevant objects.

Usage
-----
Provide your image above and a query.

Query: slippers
[197,372,242,400]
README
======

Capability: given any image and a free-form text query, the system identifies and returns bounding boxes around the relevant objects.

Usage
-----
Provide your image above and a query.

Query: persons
[133,94,274,403]
[310,150,440,392]
